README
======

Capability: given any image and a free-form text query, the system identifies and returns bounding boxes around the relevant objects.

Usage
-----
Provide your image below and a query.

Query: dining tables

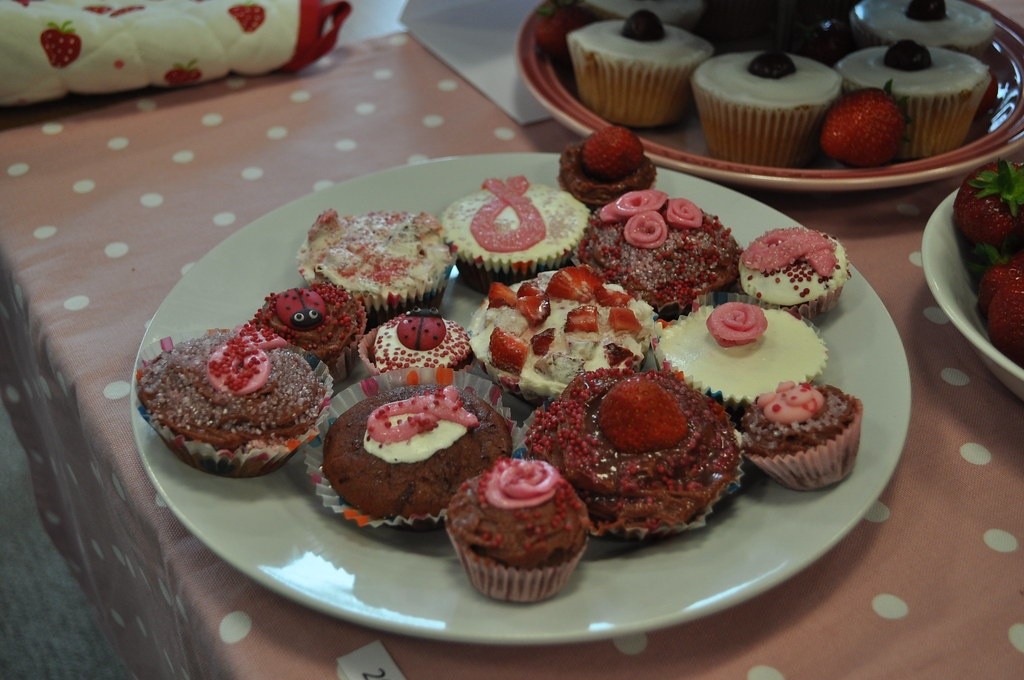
[0,30,1024,680]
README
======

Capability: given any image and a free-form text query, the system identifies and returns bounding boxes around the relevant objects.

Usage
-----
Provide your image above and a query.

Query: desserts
[567,0,996,165]
[133,144,864,602]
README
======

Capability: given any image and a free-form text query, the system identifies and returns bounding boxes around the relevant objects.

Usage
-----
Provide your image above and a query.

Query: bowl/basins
[921,188,1024,403]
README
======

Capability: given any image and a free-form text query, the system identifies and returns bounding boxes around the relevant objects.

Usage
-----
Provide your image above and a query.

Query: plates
[129,148,914,646]
[512,0,1024,193]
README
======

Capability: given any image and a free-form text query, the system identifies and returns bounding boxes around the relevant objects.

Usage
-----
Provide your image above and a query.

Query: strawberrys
[983,75,998,109]
[536,8,580,70]
[598,375,688,452]
[581,127,643,178]
[820,79,907,166]
[954,158,1024,370]
